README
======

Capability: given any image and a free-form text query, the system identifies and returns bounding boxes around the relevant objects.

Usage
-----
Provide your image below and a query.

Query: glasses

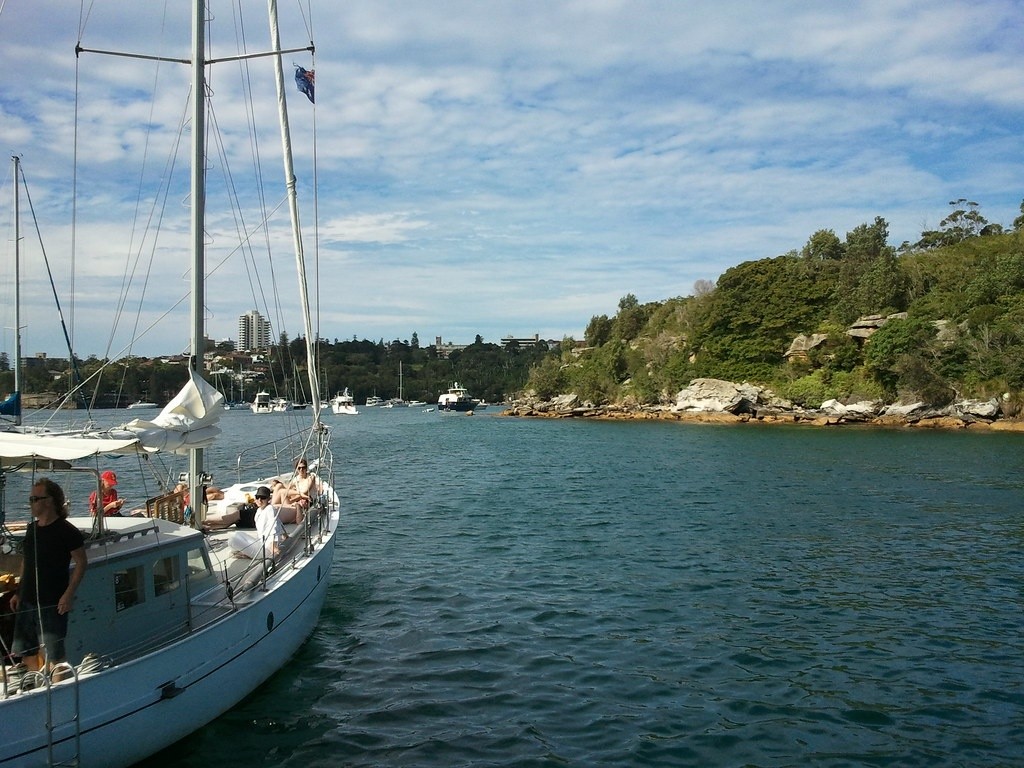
[29,496,52,502]
[255,496,268,500]
[298,466,307,470]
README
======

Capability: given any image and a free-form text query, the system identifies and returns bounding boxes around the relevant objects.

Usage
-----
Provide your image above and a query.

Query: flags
[292,62,315,104]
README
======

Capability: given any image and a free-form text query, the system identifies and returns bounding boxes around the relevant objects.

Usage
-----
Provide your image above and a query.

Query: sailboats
[320,368,329,409]
[291,359,308,410]
[386,360,409,408]
[1,1,341,768]
[215,362,252,410]
[0,153,100,436]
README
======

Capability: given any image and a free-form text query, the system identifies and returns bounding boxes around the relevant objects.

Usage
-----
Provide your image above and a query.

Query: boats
[250,389,273,414]
[366,396,386,407]
[471,399,488,411]
[126,400,159,409]
[409,401,427,407]
[273,399,293,412]
[438,381,480,411]
[331,387,359,415]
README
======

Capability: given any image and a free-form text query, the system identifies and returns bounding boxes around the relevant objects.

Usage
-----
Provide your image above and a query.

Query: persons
[8,477,87,694]
[89,470,128,517]
[172,483,190,522]
[228,486,288,560]
[199,458,316,528]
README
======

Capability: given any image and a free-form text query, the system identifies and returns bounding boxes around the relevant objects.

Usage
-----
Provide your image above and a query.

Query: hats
[254,486,271,497]
[103,471,118,486]
